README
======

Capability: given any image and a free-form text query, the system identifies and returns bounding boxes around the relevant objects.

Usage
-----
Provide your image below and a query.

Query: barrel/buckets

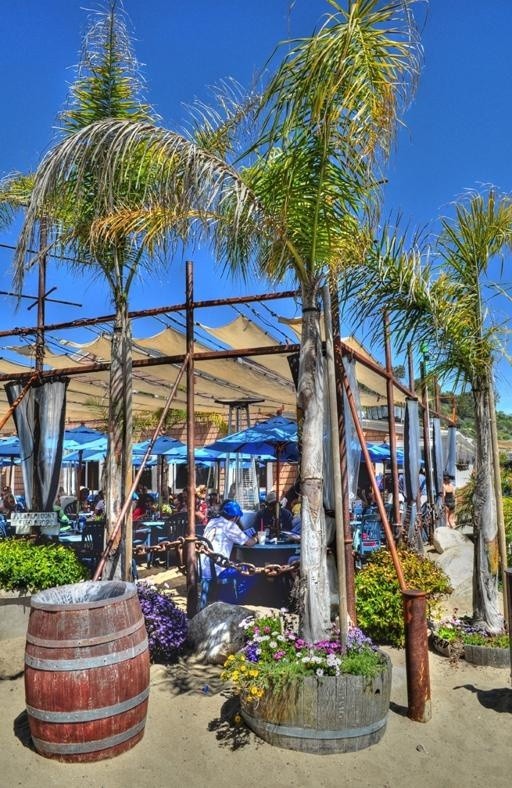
[23,579,149,761]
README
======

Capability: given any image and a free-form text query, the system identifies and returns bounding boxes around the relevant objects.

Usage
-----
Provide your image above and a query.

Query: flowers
[217,607,389,725]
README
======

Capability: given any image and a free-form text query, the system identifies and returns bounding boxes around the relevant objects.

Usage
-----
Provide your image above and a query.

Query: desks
[237,542,300,561]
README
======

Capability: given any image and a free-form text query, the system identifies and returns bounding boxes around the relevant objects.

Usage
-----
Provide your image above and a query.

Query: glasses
[265,500,276,506]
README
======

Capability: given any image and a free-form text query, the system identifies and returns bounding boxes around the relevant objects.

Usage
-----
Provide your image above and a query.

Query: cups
[257,531,266,545]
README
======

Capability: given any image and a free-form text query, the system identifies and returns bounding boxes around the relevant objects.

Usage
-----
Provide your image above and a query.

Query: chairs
[178,536,238,602]
[80,520,104,566]
[350,498,394,565]
[147,511,189,568]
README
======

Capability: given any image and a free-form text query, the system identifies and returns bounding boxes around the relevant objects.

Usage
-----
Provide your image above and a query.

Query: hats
[264,491,277,503]
[222,501,243,518]
